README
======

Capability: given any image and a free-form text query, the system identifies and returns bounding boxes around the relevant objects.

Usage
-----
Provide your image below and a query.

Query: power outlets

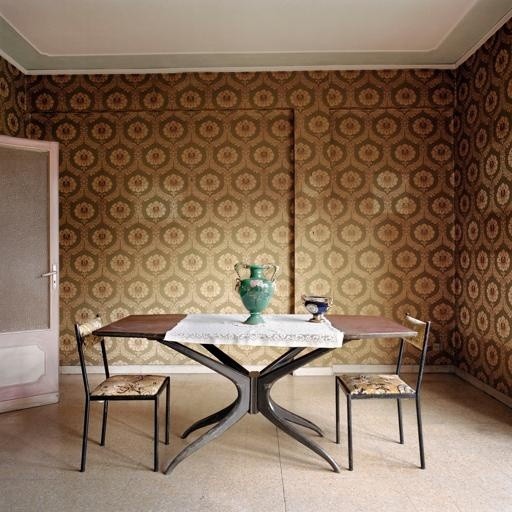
[433,343,440,353]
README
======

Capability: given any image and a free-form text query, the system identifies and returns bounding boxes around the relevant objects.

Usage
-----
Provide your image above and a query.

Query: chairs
[335,312,432,471]
[74,313,170,473]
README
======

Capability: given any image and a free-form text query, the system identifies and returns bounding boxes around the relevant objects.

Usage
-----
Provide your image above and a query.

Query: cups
[301,294,334,323]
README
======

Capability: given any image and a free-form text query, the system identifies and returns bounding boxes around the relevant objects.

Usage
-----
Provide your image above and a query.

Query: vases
[233,263,277,324]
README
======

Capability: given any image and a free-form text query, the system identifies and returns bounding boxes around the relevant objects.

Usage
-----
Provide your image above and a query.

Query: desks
[92,314,418,475]
